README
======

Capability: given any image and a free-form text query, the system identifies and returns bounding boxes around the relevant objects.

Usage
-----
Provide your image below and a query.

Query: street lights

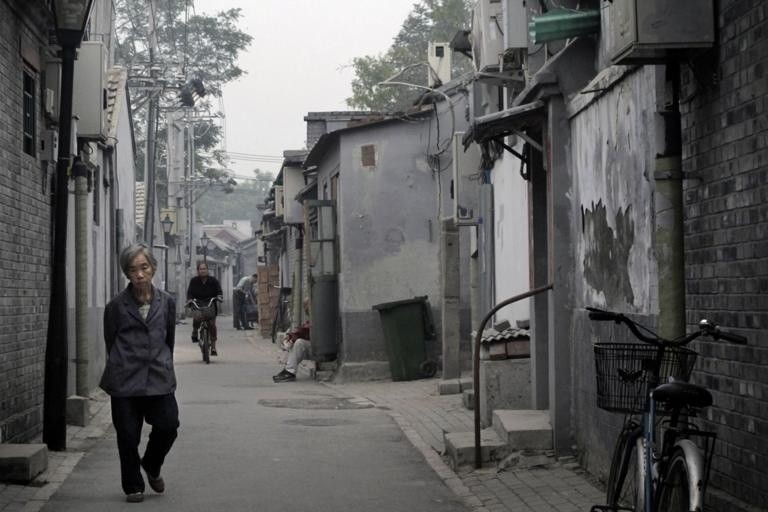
[43,0,92,453]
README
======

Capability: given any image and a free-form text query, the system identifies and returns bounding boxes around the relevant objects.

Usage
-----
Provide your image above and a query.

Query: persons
[98,240,185,504]
[186,261,225,356]
[272,296,313,384]
[232,273,258,331]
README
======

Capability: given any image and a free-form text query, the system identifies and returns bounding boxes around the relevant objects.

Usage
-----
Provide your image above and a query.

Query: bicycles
[184,296,224,363]
[267,283,291,344]
[586,307,748,512]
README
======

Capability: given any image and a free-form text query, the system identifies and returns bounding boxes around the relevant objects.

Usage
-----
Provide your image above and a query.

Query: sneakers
[141,457,165,493]
[236,326,254,329]
[126,492,145,503]
[192,336,198,341]
[273,368,296,383]
[211,351,217,356]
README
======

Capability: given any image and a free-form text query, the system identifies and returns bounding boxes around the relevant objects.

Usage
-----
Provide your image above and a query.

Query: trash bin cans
[372,295,437,381]
